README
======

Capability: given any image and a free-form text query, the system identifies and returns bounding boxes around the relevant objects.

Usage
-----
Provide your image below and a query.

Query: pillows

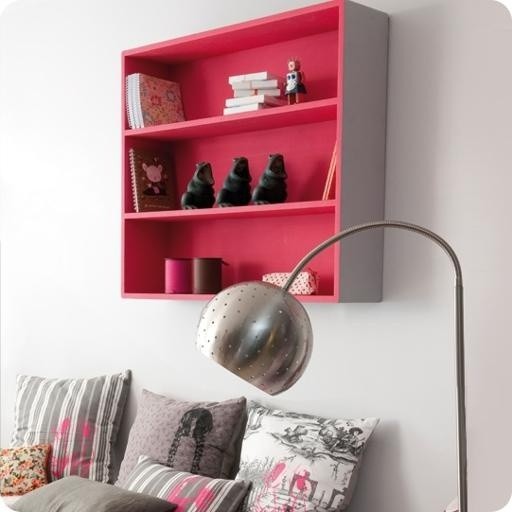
[233,399,379,511]
[3,443,51,499]
[113,386,246,484]
[6,474,179,511]
[119,454,252,511]
[7,370,132,486]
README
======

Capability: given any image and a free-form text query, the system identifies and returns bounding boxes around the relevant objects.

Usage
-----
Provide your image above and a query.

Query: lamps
[194,219,468,512]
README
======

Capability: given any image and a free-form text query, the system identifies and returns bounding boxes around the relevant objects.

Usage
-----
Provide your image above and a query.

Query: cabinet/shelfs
[121,0,389,303]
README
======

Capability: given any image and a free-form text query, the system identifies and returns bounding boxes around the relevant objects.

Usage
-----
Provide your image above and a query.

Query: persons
[282,60,307,107]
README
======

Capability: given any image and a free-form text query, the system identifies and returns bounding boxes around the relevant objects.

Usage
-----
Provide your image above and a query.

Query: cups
[193,256,222,295]
[163,255,194,294]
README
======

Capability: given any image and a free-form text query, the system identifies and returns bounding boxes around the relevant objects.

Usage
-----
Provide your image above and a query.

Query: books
[321,138,336,202]
[128,146,184,214]
[324,148,336,204]
[221,71,288,115]
[124,71,185,131]
